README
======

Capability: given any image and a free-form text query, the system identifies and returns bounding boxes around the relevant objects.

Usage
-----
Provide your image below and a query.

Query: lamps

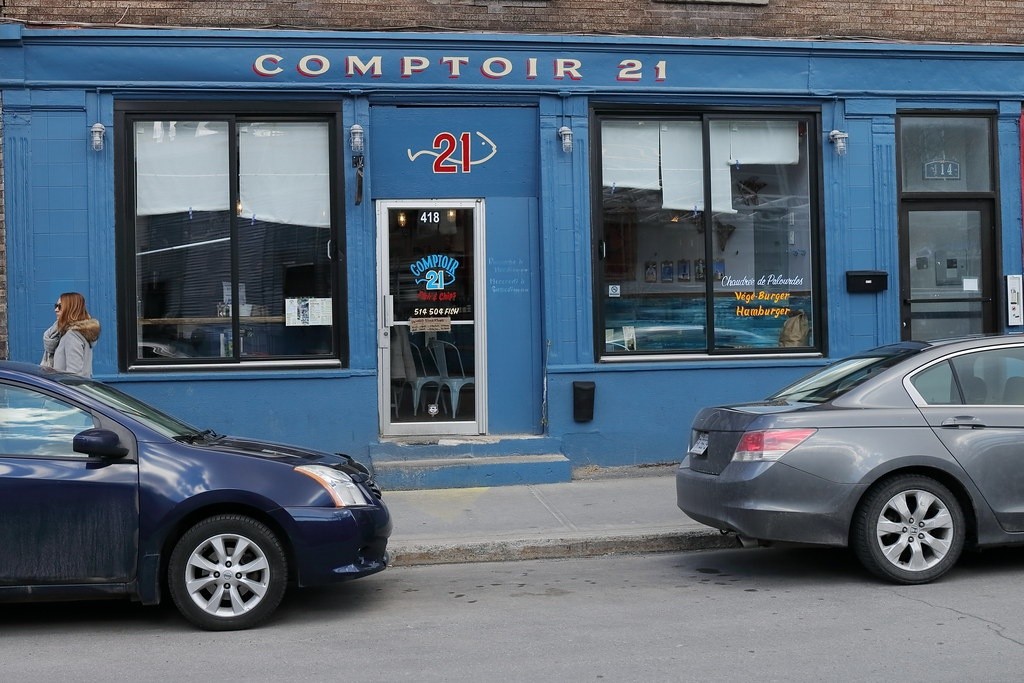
[351,124,365,153]
[90,123,105,150]
[559,125,574,154]
[830,130,848,155]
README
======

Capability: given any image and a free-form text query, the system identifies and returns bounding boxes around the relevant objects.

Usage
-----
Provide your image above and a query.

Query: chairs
[391,340,475,420]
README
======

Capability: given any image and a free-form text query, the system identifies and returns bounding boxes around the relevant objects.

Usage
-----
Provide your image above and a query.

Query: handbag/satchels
[40,350,54,367]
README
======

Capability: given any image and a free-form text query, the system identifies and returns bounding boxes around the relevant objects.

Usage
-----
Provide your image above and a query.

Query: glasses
[55,304,61,310]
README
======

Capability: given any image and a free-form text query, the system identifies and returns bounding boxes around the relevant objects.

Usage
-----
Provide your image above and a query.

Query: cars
[674,332,1024,586]
[0,356,395,635]
[604,320,780,352]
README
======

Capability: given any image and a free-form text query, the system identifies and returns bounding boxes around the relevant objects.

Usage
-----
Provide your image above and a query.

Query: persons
[38,291,101,428]
